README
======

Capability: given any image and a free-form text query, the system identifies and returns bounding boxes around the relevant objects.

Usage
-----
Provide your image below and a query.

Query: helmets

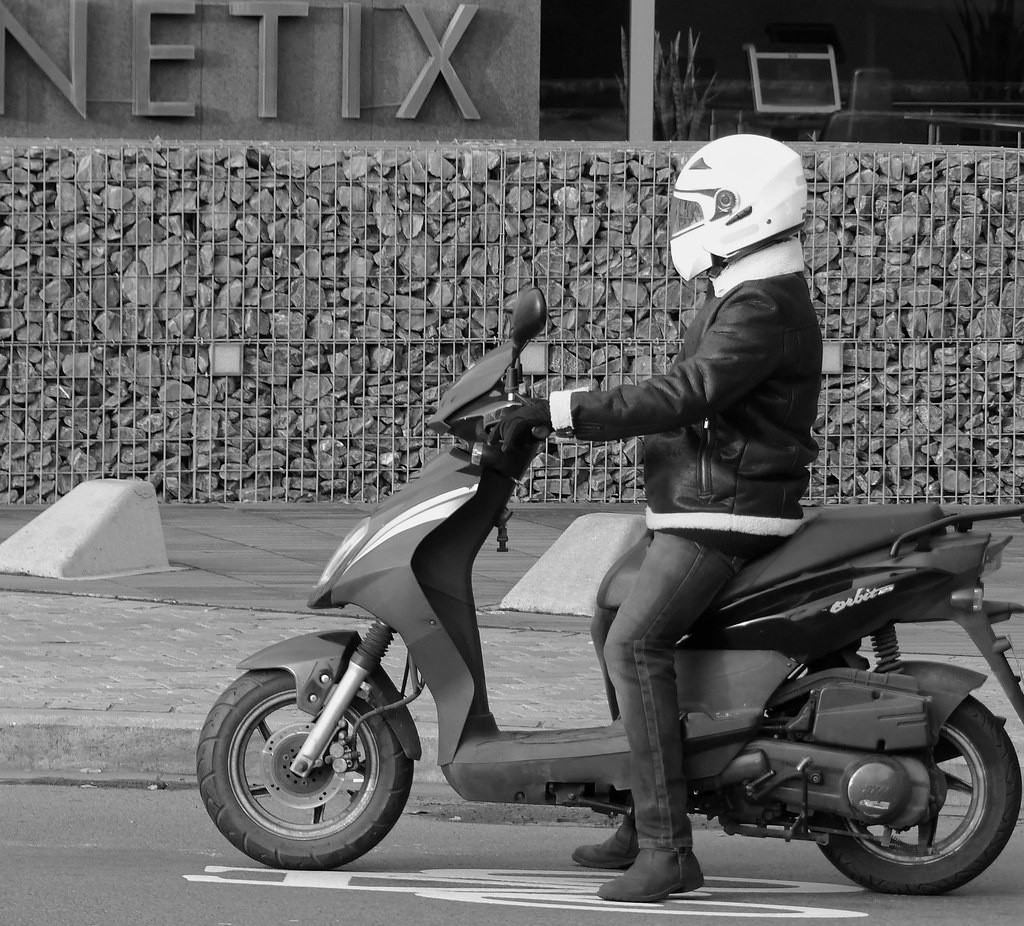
[668,133,809,283]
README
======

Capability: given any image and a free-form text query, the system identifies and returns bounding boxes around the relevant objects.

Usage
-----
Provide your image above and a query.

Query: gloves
[486,397,550,453]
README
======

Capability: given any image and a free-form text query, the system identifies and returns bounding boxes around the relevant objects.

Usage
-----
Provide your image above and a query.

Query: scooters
[197,288,1024,895]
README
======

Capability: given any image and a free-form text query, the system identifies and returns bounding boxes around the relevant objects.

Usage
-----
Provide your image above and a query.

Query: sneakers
[595,849,705,902]
[571,823,640,867]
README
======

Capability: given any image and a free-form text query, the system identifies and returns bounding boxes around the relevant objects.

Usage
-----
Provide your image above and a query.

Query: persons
[486,134,824,901]
